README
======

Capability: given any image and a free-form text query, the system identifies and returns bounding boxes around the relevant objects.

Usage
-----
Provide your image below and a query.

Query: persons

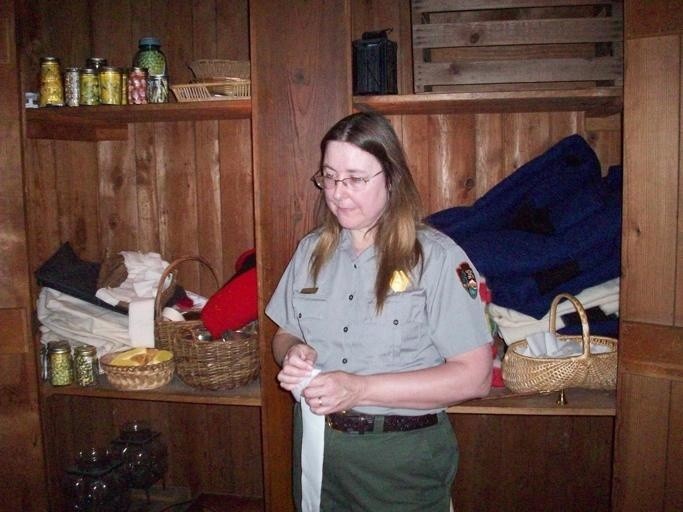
[261,108,495,512]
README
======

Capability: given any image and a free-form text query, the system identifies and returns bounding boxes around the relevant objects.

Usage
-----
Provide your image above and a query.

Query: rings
[315,395,323,408]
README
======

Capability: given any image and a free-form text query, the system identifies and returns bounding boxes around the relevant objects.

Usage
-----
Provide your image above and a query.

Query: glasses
[312,169,384,191]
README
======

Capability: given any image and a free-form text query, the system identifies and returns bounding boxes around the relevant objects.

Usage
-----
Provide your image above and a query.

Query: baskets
[102,261,178,314]
[170,78,250,102]
[187,60,250,78]
[172,325,260,390]
[154,256,219,349]
[503,292,618,394]
[99,347,174,391]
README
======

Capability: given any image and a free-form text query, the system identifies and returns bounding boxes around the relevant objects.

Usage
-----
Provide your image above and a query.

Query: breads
[110,346,172,368]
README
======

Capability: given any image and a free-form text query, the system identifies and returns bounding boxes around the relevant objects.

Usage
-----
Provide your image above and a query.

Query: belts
[326,412,436,434]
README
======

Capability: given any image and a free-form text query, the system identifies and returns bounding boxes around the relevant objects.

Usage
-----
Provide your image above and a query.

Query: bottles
[38,340,99,388]
[40,36,168,106]
[189,321,254,344]
[352,30,397,94]
[62,417,168,512]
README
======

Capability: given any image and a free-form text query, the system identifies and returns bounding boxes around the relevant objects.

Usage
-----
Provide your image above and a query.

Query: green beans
[137,51,166,75]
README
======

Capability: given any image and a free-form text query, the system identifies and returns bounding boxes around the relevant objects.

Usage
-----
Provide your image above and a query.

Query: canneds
[192,328,212,341]
[40,343,98,387]
[39,57,168,108]
